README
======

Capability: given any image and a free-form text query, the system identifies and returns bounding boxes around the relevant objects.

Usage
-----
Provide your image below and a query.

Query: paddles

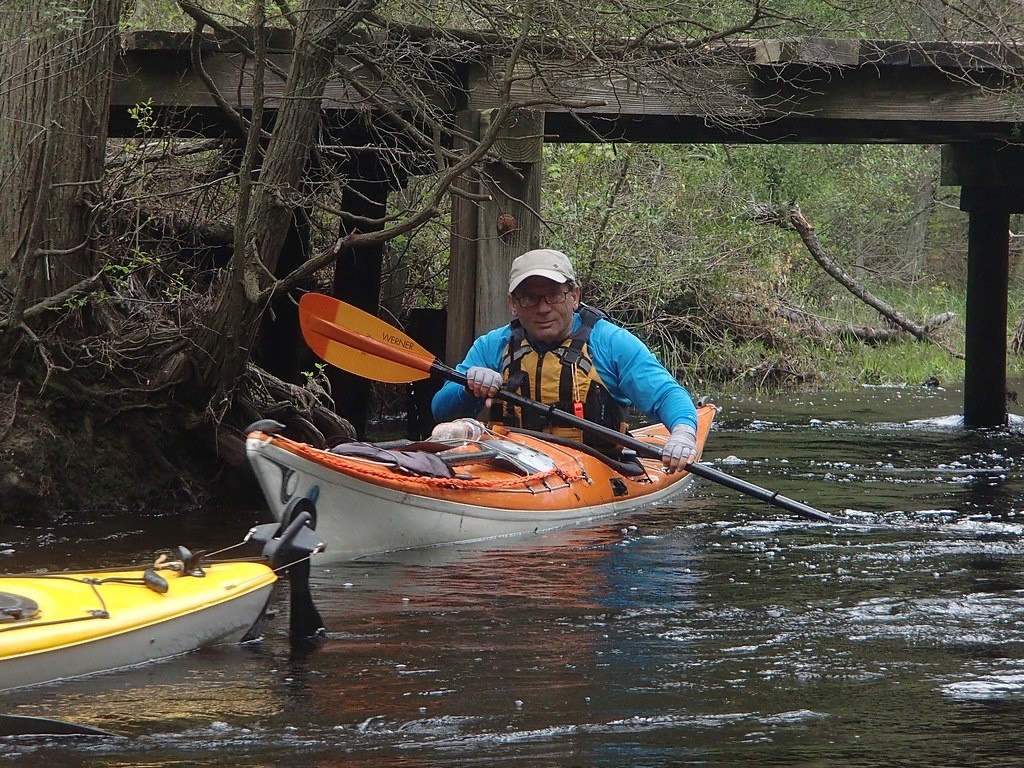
[298,291,853,524]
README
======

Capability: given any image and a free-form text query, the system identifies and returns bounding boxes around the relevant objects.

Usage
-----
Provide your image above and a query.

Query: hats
[509,249,575,293]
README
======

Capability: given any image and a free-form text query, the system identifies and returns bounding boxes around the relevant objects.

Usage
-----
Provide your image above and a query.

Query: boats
[244,403,719,563]
[1,560,279,693]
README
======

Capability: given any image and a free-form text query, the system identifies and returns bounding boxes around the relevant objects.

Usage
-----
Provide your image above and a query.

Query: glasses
[513,287,573,309]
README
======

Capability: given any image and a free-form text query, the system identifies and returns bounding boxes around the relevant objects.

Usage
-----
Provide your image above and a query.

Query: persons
[431,249,698,474]
